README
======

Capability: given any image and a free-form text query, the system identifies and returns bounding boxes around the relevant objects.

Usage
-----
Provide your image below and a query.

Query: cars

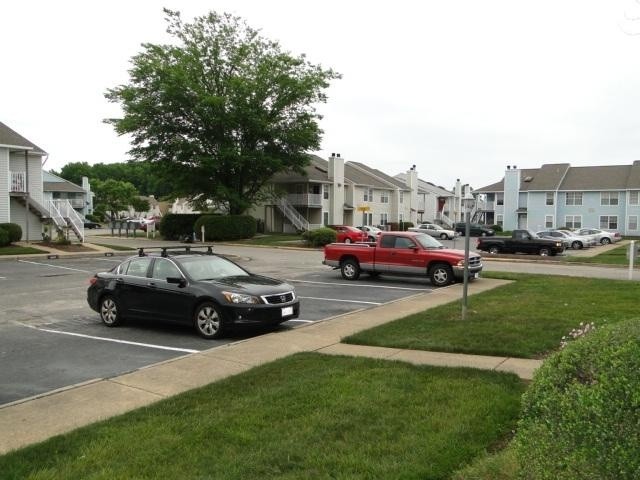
[86,245,300,341]
[356,225,383,242]
[573,227,623,245]
[535,230,595,249]
[455,222,495,237]
[407,224,459,240]
[336,225,368,243]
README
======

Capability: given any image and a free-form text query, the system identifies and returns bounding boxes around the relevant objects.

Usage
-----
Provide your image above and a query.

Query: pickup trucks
[321,229,483,287]
[475,229,564,257]
